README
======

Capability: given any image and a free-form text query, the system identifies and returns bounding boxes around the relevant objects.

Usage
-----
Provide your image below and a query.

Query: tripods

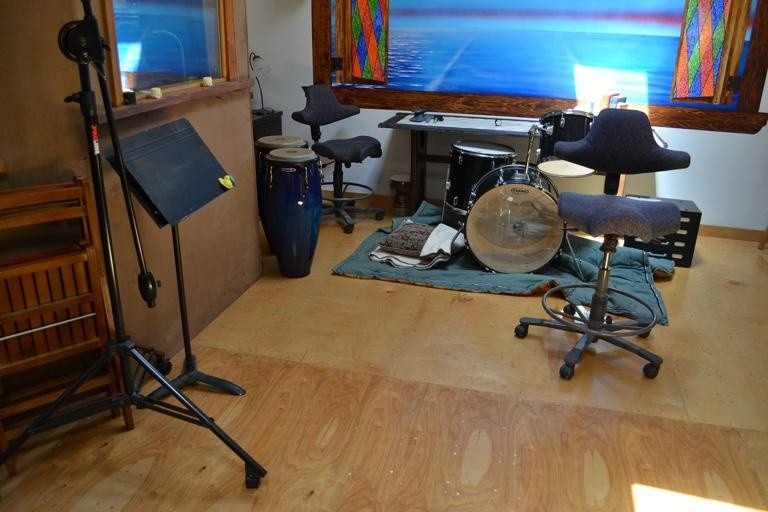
[140,223,246,412]
[0,18,267,491]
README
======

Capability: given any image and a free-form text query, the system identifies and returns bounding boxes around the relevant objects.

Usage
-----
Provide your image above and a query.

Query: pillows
[376,221,436,257]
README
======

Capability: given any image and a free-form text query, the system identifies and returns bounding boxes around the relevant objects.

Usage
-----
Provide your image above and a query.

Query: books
[594,92,628,116]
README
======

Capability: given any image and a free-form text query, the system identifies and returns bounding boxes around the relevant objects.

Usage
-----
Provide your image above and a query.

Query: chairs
[291,80,389,235]
[510,107,693,380]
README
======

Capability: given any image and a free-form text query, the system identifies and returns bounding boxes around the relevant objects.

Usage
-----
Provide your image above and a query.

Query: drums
[536,108,598,178]
[256,133,323,280]
[466,165,565,273]
[443,139,517,235]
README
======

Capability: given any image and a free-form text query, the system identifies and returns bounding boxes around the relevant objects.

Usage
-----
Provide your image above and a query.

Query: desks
[375,108,542,217]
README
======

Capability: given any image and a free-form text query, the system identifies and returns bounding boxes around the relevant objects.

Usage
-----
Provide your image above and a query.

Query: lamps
[248,47,274,116]
